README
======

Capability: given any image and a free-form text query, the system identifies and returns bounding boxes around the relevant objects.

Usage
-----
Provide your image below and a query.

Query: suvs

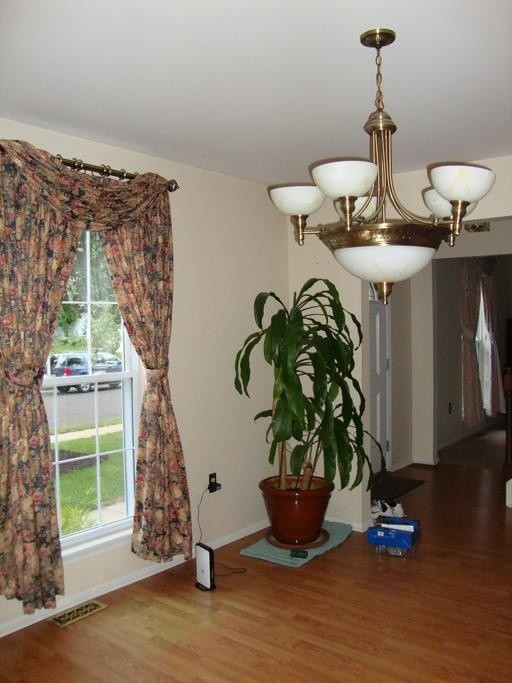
[55,350,124,392]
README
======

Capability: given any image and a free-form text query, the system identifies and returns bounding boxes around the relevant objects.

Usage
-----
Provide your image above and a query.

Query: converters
[207,482,223,493]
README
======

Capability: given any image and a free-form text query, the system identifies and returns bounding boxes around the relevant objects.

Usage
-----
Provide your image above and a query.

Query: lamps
[265,25,498,308]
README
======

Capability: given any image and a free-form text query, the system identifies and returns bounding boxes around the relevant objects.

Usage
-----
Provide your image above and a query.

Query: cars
[42,353,62,374]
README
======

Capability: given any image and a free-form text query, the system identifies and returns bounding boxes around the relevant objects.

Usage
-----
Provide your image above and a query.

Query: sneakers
[387,501,404,517]
[370,499,392,519]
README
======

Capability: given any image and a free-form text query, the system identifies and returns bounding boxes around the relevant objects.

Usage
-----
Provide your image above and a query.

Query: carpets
[239,519,354,569]
[371,473,426,502]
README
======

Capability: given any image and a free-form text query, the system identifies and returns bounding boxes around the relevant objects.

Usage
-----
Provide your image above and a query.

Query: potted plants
[232,275,389,551]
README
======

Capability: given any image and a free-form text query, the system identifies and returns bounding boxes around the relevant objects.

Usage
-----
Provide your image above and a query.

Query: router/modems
[195,542,216,591]
